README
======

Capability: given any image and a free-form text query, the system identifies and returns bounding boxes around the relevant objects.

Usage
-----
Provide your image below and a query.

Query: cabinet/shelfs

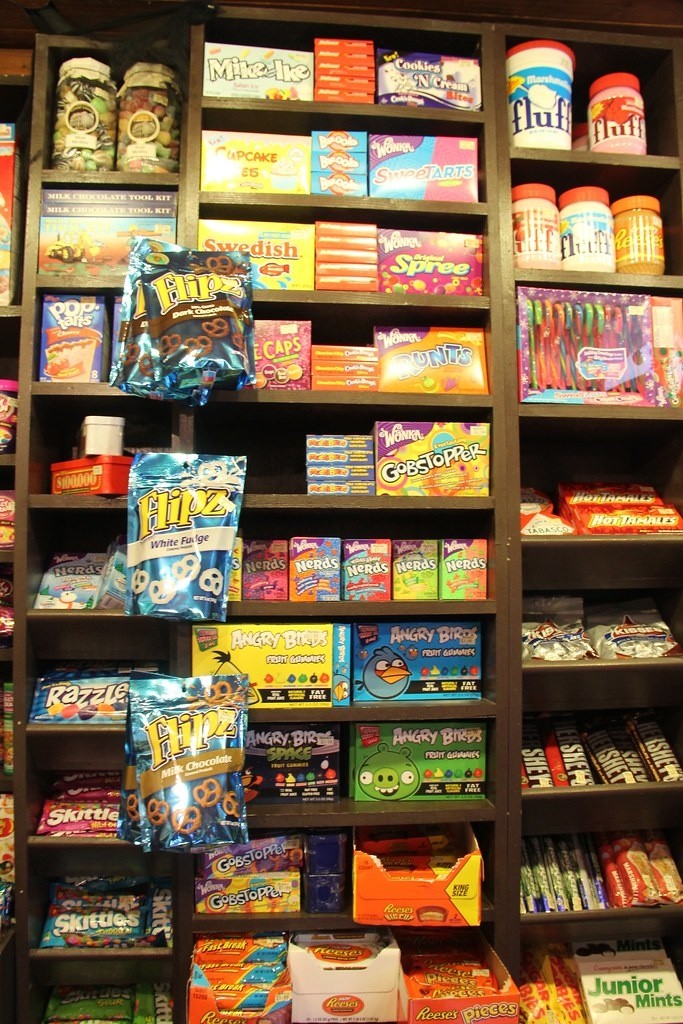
[15,13,506,1024]
[0,29,35,1024]
[495,19,682,988]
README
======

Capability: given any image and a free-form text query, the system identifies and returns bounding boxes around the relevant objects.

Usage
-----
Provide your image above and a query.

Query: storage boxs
[38,37,683,1024]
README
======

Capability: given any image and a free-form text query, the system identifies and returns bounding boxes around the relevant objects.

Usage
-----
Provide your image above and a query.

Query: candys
[54,84,181,173]
